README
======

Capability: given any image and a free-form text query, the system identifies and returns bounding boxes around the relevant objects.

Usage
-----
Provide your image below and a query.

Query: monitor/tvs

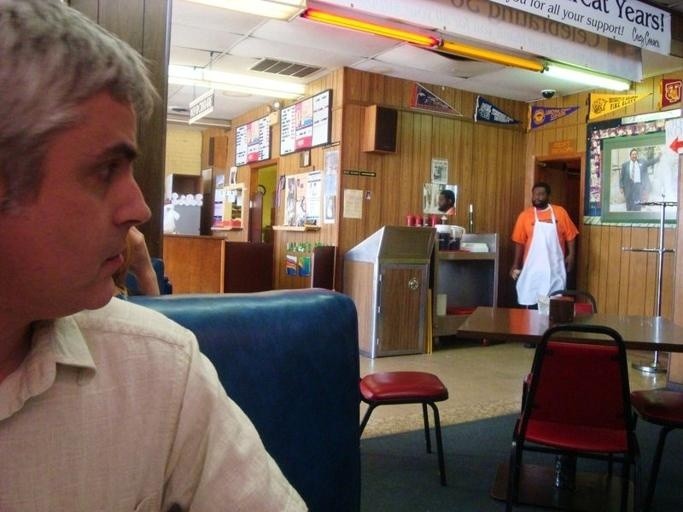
[278,88,333,157]
[234,111,272,167]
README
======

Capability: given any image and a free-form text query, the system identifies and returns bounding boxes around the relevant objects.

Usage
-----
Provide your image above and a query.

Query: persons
[619,148,662,212]
[286,180,294,208]
[112,226,160,296]
[1,0,309,512]
[437,189,456,216]
[509,182,581,349]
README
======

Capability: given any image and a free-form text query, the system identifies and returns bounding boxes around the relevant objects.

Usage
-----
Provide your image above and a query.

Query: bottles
[466,204,475,233]
[406,211,437,227]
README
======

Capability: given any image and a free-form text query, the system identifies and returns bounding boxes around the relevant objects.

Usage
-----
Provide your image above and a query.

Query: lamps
[300,8,441,50]
[438,40,546,75]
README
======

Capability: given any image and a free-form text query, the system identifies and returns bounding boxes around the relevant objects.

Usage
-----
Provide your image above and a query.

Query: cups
[436,223,464,251]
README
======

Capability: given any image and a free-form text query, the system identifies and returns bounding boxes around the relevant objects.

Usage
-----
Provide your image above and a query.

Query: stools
[626,386,683,512]
[354,370,452,486]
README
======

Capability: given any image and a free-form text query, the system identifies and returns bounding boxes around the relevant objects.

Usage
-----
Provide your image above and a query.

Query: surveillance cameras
[543,91,554,98]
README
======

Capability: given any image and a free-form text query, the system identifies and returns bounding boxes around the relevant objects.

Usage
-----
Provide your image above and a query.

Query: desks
[453,304,683,511]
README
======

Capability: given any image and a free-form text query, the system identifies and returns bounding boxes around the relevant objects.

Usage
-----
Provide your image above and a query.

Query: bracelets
[512,264,520,268]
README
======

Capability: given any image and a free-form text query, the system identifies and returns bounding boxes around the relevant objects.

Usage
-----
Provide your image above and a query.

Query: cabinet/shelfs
[342,256,427,360]
[210,182,250,231]
[431,232,501,352]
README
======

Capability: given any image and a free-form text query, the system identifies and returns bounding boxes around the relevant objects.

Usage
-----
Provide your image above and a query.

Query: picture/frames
[597,132,677,226]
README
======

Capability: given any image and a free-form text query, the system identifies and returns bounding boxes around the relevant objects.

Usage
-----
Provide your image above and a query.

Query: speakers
[362,104,400,154]
[311,245,337,291]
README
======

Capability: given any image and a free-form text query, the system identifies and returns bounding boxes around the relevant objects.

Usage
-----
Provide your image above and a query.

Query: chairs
[120,285,362,512]
[505,289,649,509]
